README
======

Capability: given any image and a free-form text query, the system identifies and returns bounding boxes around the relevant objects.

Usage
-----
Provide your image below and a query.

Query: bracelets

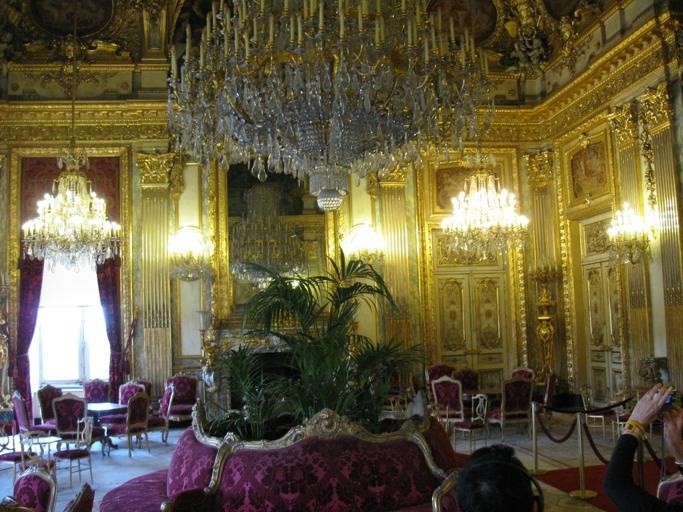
[624,419,650,442]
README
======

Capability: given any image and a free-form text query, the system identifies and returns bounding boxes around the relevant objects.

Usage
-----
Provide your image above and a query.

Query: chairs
[14,378,150,458]
[425,364,557,452]
[0,417,95,512]
[657,471,682,505]
[583,393,615,440]
[617,390,652,441]
[150,372,197,441]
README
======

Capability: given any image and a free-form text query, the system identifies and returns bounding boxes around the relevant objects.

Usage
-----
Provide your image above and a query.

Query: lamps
[346,246,384,270]
[603,199,658,266]
[431,130,529,268]
[18,0,121,272]
[159,1,494,210]
[165,249,212,283]
[228,178,312,281]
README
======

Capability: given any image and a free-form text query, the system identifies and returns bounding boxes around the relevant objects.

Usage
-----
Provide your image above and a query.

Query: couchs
[100,405,467,512]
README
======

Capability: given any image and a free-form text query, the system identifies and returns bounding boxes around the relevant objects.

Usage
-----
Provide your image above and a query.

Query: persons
[603,384,683,512]
[455,444,537,512]
[172,488,224,512]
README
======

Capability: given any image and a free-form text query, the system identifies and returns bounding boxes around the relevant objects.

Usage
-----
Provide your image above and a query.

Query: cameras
[651,390,673,410]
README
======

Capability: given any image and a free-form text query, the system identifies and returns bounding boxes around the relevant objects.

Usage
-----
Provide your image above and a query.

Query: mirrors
[206,156,340,327]
